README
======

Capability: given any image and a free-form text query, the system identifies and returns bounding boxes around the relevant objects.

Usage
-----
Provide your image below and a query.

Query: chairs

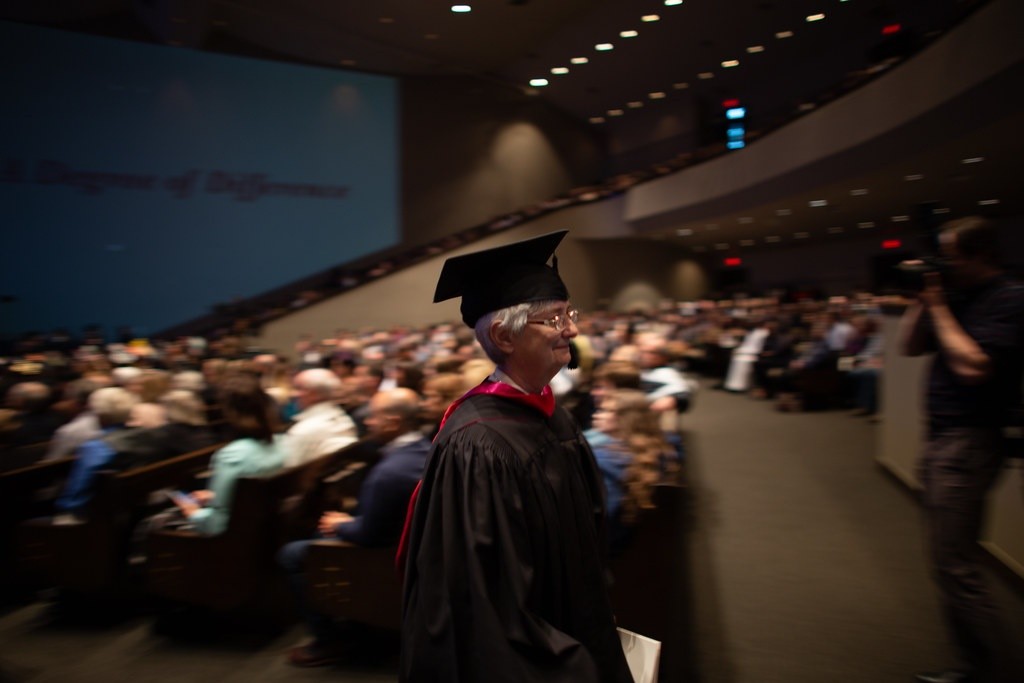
[0,435,682,634]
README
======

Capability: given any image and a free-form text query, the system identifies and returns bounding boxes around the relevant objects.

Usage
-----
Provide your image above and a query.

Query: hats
[433,228,570,329]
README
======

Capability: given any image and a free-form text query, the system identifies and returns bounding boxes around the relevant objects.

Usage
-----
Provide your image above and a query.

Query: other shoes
[290,641,339,665]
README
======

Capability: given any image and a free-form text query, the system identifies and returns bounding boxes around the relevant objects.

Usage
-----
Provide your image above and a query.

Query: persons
[900,217,1024,683]
[395,230,635,683]
[0,288,917,666]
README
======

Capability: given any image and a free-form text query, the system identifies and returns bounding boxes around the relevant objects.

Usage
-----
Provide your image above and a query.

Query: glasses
[526,309,579,332]
